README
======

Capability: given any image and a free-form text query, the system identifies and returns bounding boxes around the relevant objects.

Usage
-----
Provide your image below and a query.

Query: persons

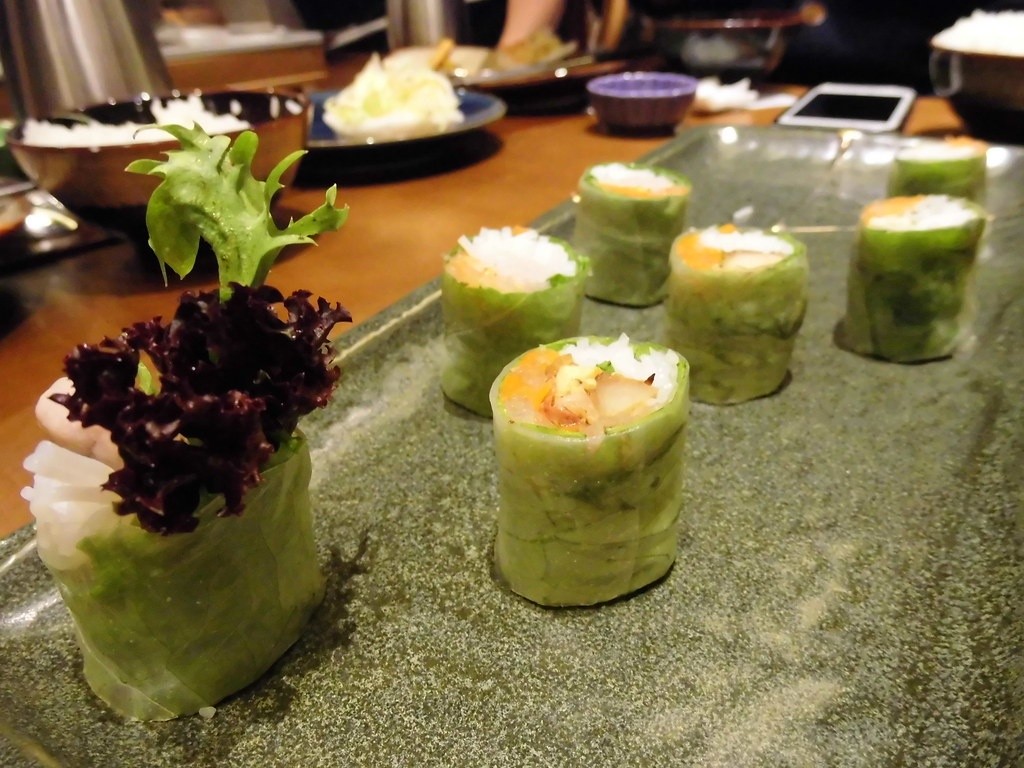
[498,1,847,89]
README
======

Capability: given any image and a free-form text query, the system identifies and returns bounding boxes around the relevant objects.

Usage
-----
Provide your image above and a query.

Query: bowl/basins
[587,73,698,131]
[652,11,798,79]
[5,86,310,247]
[930,34,1024,143]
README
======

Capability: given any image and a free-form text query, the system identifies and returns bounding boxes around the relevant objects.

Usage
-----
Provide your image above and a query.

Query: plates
[306,88,508,170]
[472,58,624,104]
[1,122,1024,766]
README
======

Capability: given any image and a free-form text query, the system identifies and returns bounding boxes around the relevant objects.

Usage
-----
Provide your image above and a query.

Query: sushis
[440,134,988,607]
[20,125,356,723]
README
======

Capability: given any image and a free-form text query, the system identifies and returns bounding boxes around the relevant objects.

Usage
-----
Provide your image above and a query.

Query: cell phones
[778,82,916,135]
